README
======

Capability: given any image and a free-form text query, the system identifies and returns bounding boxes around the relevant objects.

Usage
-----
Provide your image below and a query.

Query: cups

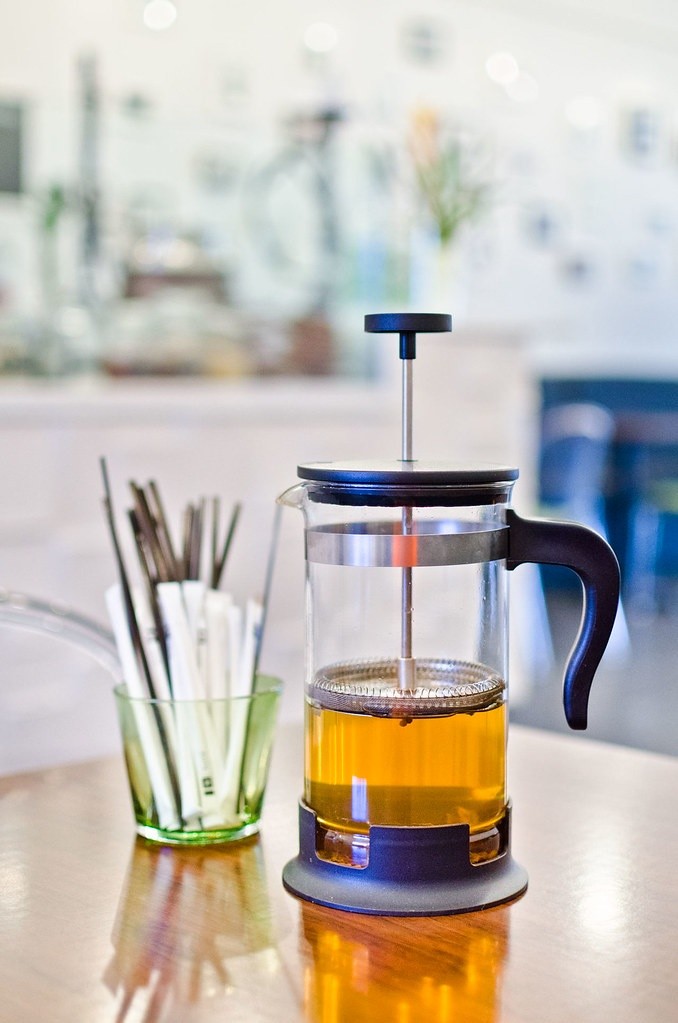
[112,674,286,847]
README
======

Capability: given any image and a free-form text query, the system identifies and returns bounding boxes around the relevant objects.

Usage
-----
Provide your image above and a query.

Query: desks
[0,713,678,1023]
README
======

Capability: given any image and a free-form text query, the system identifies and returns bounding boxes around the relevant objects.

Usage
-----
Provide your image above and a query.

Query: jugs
[272,312,623,916]
